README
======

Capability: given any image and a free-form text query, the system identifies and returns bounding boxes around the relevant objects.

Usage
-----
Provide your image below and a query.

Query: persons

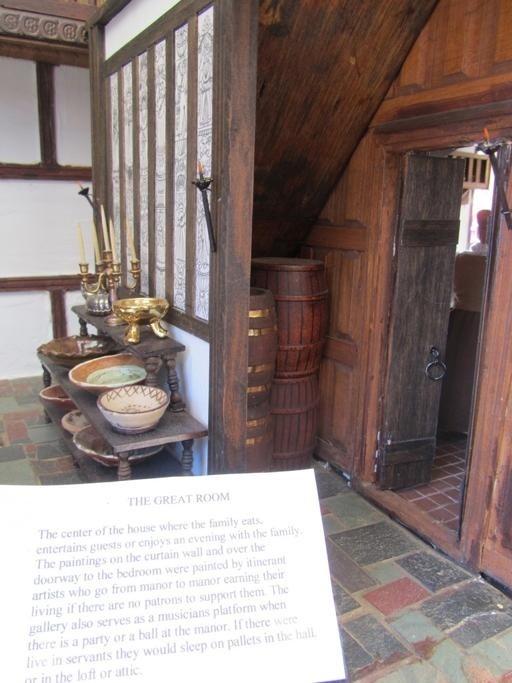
[471,209,492,252]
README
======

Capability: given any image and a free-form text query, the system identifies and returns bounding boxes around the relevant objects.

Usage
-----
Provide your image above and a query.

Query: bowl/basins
[36,330,170,469]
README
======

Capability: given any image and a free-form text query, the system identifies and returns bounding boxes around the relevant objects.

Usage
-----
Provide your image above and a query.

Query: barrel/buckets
[248,285,277,408]
[270,371,322,470]
[247,401,276,472]
[250,256,329,377]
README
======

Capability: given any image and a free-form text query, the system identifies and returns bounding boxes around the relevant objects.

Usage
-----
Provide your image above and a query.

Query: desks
[40,355,207,480]
[71,305,185,412]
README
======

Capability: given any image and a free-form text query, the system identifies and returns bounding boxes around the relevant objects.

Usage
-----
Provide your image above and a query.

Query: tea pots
[84,284,111,317]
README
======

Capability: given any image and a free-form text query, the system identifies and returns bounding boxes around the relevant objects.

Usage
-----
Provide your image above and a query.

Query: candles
[128,223,137,260]
[90,221,101,264]
[76,223,85,265]
[109,219,116,262]
[100,204,110,250]
